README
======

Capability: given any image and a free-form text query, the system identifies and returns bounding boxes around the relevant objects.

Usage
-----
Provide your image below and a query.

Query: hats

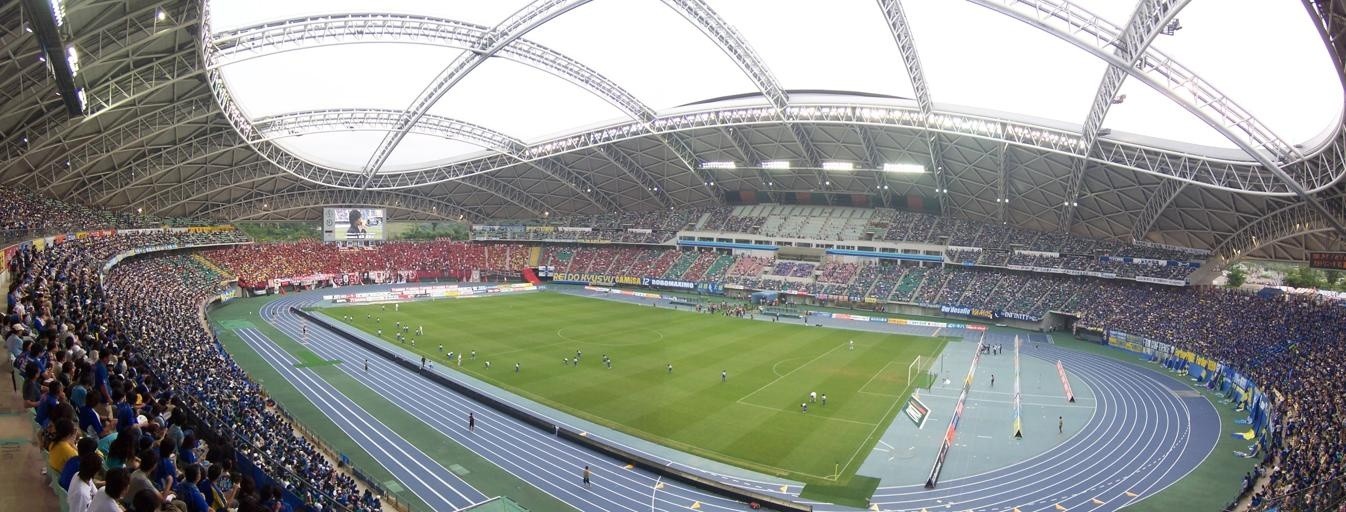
[12,323,25,330]
[152,405,165,414]
[107,361,115,366]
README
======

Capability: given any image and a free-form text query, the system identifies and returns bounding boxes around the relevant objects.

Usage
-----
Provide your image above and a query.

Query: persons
[0,192,1345,512]
